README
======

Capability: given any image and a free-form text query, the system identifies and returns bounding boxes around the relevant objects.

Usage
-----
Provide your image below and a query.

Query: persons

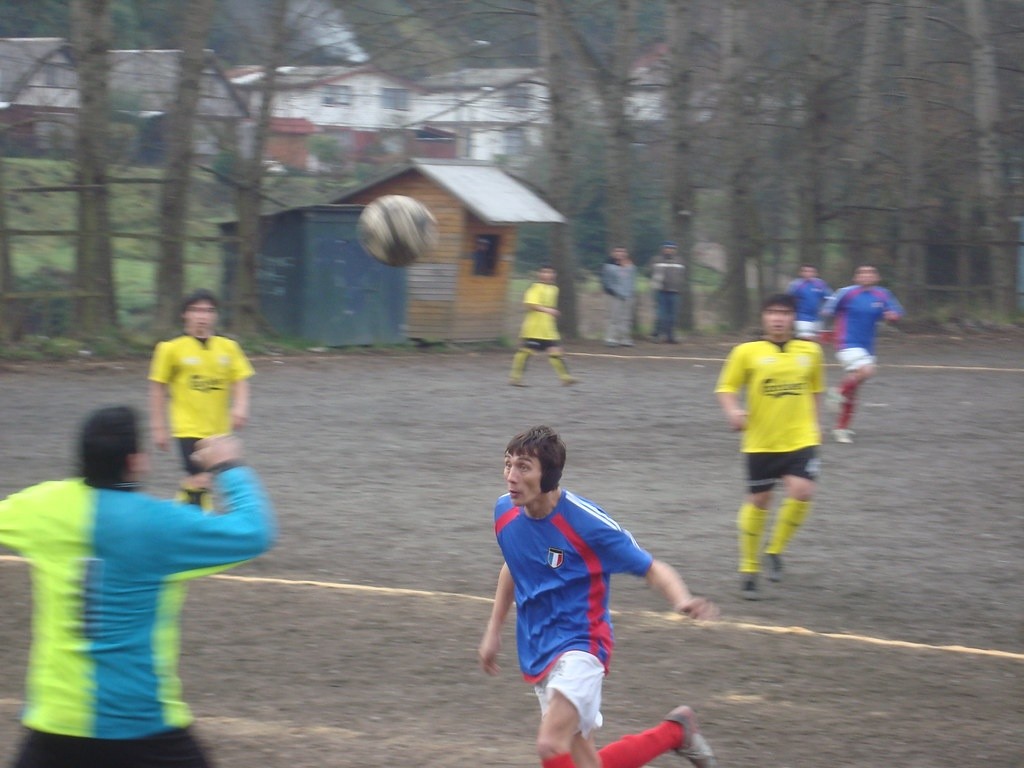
[715,294,829,602]
[601,246,642,346]
[148,284,256,514]
[783,265,907,444]
[510,265,581,388]
[649,239,685,344]
[479,424,716,768]
[0,407,281,768]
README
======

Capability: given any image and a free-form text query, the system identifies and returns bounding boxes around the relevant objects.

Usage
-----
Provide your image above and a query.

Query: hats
[660,239,682,249]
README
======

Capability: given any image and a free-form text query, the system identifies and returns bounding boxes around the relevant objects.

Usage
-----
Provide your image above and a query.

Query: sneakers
[665,705,718,768]
[831,428,855,444]
[827,387,847,403]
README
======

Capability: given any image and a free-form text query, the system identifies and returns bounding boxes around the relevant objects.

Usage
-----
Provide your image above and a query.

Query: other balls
[360,191,434,266]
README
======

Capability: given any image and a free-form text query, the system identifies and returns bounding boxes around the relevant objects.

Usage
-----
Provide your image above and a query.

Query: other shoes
[606,341,616,347]
[505,377,531,386]
[737,576,764,600]
[621,342,633,347]
[561,375,578,385]
[666,338,680,345]
[762,553,786,582]
